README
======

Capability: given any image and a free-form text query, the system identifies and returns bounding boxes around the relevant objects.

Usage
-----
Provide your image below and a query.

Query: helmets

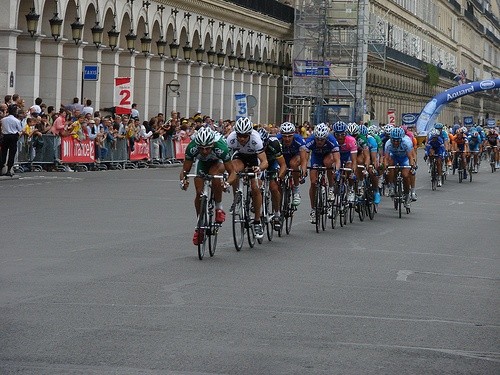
[348,122,360,135]
[367,124,408,139]
[234,116,253,134]
[434,123,443,129]
[428,129,440,140]
[332,121,347,132]
[358,124,369,136]
[257,127,271,143]
[313,122,330,139]
[194,126,216,149]
[279,122,296,137]
[452,122,495,134]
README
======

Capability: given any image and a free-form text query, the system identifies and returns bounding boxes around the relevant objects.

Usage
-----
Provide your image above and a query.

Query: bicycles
[182,169,228,261]
[426,145,499,191]
[260,165,303,242]
[306,162,415,234]
[222,163,266,252]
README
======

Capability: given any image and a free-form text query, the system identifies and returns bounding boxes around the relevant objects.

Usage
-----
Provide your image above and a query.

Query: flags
[453,69,466,81]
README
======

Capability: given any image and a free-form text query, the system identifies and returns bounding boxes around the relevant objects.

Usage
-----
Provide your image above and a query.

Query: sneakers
[215,209,226,222]
[192,228,204,246]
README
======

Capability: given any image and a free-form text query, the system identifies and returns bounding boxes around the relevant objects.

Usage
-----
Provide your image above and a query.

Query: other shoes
[229,200,241,214]
[428,154,500,187]
[253,223,264,240]
[0,170,3,176]
[270,177,418,232]
[6,172,13,176]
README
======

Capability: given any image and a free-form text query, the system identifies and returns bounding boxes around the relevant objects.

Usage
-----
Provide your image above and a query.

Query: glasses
[335,133,346,137]
[237,134,250,138]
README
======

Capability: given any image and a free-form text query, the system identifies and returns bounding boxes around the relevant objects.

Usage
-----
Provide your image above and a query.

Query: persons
[0,94,500,230]
[180,126,237,245]
[226,117,264,239]
